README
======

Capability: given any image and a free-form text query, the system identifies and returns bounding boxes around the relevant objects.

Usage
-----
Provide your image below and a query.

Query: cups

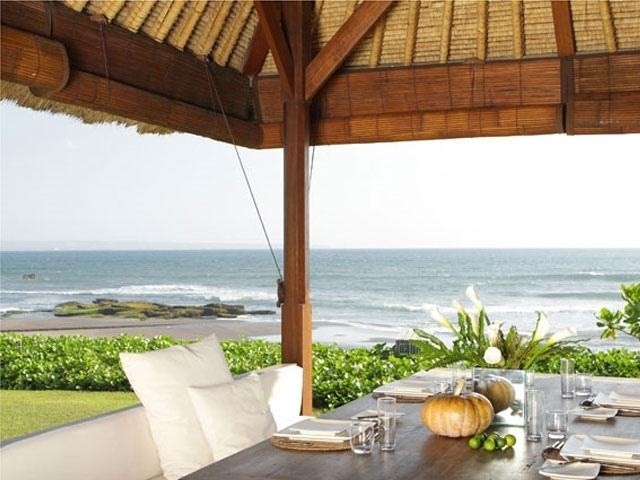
[524,370,535,386]
[524,390,543,442]
[561,358,575,398]
[546,410,569,438]
[377,396,397,451]
[351,422,374,455]
[578,374,594,395]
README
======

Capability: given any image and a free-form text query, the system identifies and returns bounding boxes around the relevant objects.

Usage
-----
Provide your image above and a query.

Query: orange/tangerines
[469,432,516,452]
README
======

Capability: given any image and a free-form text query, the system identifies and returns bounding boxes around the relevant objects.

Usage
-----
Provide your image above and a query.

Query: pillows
[117,333,233,480]
[188,368,277,462]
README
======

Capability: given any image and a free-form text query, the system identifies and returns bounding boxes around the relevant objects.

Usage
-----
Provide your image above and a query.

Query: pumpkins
[474,374,515,413]
[421,379,495,438]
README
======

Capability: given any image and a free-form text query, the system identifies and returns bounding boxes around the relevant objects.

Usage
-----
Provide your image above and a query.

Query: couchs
[0,361,317,479]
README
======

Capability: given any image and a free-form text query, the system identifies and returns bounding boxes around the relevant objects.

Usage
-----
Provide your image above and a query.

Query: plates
[274,408,405,443]
[539,433,640,479]
[572,391,640,422]
[373,379,448,400]
